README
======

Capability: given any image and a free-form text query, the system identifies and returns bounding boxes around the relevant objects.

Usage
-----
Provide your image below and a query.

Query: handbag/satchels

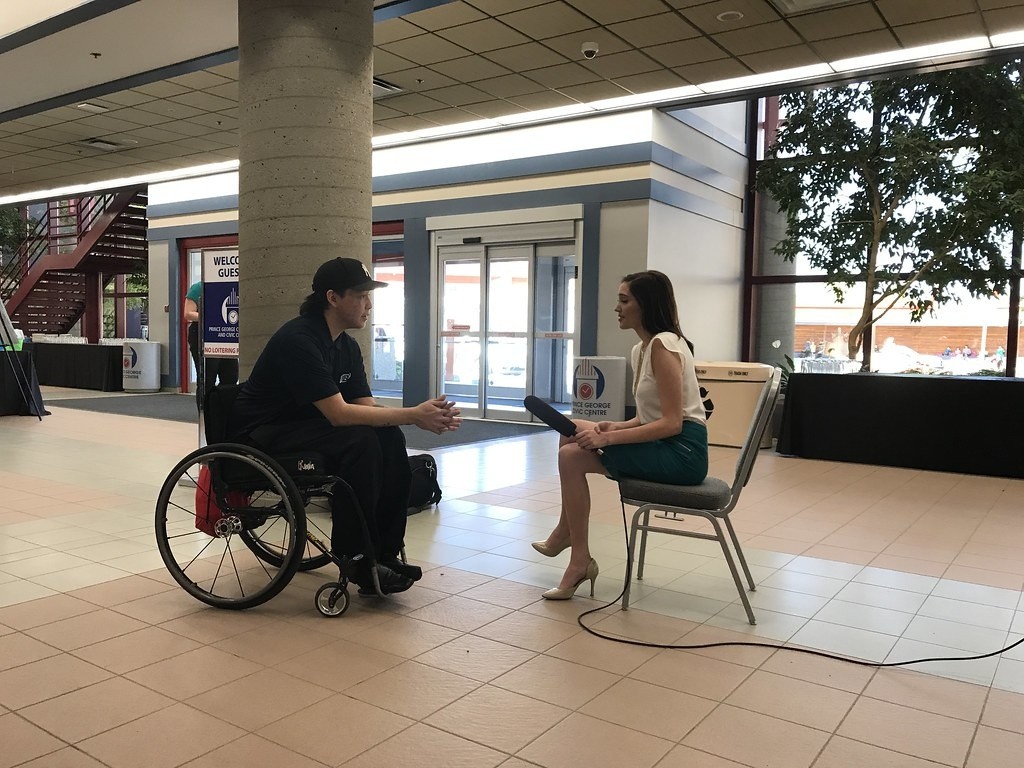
[196,465,252,538]
[405,454,442,512]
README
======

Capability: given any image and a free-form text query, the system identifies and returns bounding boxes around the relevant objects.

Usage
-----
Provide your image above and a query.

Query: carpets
[43,394,554,451]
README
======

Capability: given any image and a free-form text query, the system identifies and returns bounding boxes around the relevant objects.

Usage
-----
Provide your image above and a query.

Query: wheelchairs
[153,381,407,619]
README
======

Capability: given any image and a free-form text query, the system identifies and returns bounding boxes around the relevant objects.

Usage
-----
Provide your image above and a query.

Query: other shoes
[375,555,422,581]
[358,563,414,594]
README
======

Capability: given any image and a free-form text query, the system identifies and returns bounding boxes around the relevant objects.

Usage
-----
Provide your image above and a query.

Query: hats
[315,257,388,292]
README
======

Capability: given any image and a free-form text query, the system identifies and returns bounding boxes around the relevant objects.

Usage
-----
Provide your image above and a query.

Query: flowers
[772,340,795,394]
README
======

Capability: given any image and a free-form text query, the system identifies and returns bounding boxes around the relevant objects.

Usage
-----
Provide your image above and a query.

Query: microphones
[524,395,607,457]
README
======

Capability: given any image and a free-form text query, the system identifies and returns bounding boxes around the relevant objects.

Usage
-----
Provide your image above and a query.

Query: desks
[0,351,51,416]
[775,371,1024,480]
[21,342,125,393]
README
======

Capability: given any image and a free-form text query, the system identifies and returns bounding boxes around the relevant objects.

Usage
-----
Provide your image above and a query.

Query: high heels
[531,534,573,557]
[543,558,598,600]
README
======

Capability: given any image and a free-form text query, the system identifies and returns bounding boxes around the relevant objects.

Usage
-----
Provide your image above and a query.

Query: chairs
[619,367,782,625]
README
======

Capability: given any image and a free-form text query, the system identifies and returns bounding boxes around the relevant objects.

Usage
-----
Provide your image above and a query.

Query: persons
[806,338,811,359]
[942,345,951,358]
[184,280,239,413]
[225,258,463,595]
[532,270,708,600]
[962,345,971,357]
[954,347,961,356]
[995,345,1005,357]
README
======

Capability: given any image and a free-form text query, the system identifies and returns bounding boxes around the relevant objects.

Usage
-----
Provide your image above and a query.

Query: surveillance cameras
[582,42,599,59]
